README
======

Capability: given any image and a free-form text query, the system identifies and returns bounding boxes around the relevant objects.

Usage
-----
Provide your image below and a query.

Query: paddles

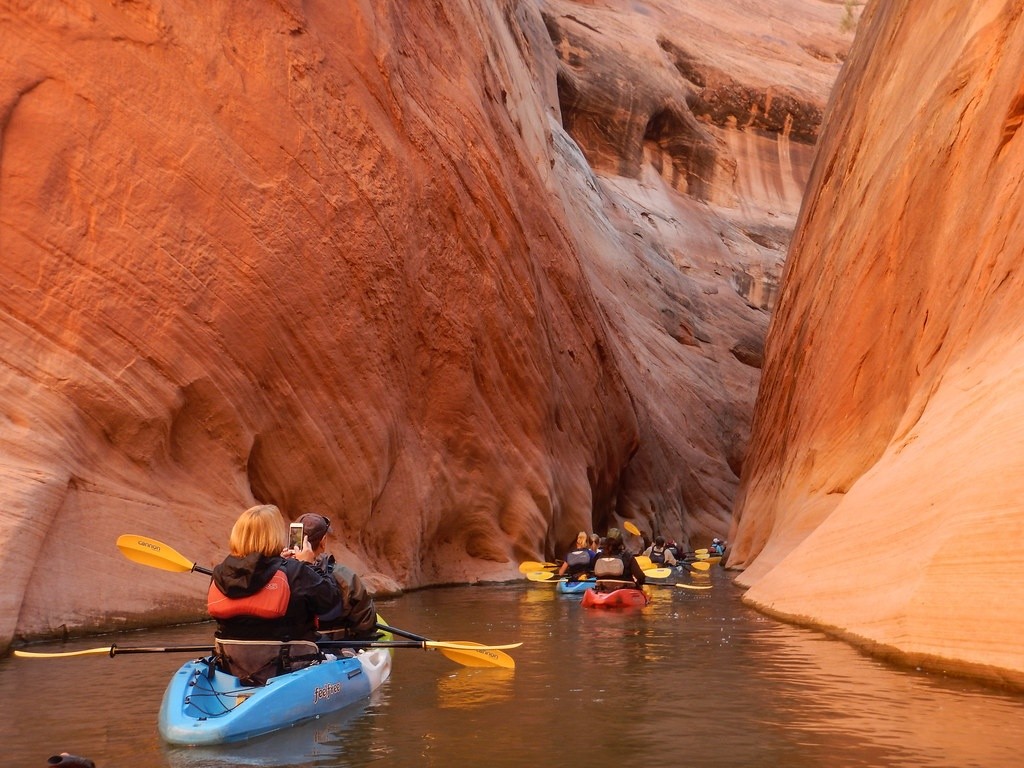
[114,532,517,678]
[11,637,525,662]
[517,555,713,589]
[621,520,721,571]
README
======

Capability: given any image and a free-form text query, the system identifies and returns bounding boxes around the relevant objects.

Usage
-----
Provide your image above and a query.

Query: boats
[158,613,395,746]
[581,585,652,608]
[637,554,723,583]
[556,574,599,595]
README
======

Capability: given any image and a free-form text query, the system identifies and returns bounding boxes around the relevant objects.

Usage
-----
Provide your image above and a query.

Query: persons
[560,530,651,581]
[642,536,676,568]
[709,537,727,557]
[663,537,686,563]
[208,505,344,680]
[584,528,646,594]
[291,513,386,657]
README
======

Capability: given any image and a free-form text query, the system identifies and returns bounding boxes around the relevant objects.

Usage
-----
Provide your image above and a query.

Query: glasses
[321,516,330,529]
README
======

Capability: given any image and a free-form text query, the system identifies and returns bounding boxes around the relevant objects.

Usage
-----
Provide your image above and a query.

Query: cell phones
[289,523,303,553]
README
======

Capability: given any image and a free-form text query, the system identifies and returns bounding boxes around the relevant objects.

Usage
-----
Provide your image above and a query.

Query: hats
[655,535,665,545]
[607,528,621,540]
[713,538,720,543]
[294,513,330,541]
